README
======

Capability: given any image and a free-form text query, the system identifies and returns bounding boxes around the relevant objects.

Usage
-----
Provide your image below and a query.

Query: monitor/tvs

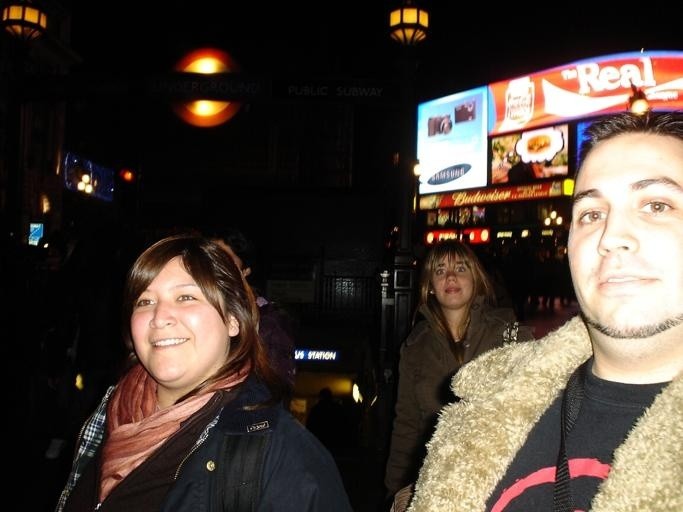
[28,221,45,247]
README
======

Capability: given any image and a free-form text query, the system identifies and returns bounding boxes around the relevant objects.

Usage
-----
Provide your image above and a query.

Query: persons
[200,224,298,418]
[389,108,682,510]
[385,238,537,500]
[51,232,354,512]
[471,241,575,323]
[305,385,352,461]
